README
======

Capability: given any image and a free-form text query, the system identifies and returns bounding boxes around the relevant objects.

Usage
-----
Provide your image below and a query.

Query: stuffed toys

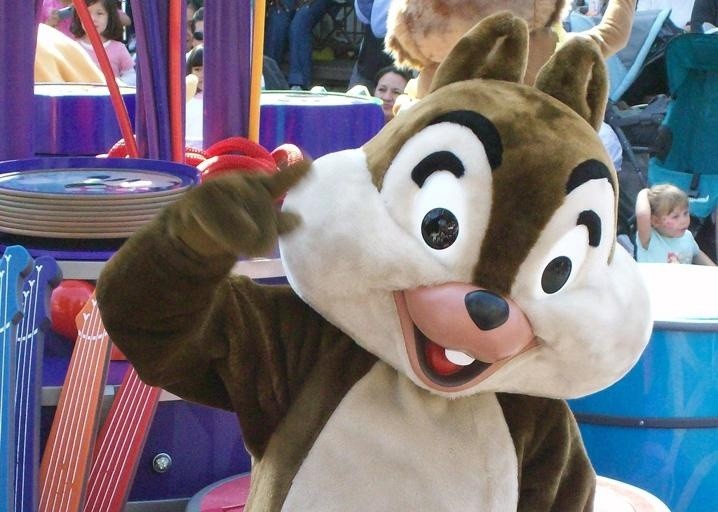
[95,13,658,512]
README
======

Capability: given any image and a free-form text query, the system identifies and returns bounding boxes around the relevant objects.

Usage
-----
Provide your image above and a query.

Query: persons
[34,0,420,154]
[558,0,716,265]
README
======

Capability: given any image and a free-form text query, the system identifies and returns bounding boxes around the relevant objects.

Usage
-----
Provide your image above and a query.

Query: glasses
[193,31,203,41]
